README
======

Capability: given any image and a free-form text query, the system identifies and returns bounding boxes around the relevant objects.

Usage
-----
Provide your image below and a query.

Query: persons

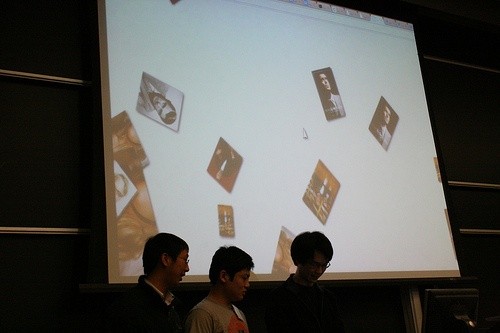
[214,148,237,180]
[317,70,345,119]
[137,73,177,125]
[103,232,189,333]
[370,104,393,147]
[264,231,344,333]
[187,246,254,332]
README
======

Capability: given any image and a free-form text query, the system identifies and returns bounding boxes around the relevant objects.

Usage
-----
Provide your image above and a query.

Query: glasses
[171,256,189,264]
[302,261,330,269]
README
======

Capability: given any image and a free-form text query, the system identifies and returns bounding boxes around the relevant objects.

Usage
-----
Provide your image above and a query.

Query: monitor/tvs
[421,288,479,333]
